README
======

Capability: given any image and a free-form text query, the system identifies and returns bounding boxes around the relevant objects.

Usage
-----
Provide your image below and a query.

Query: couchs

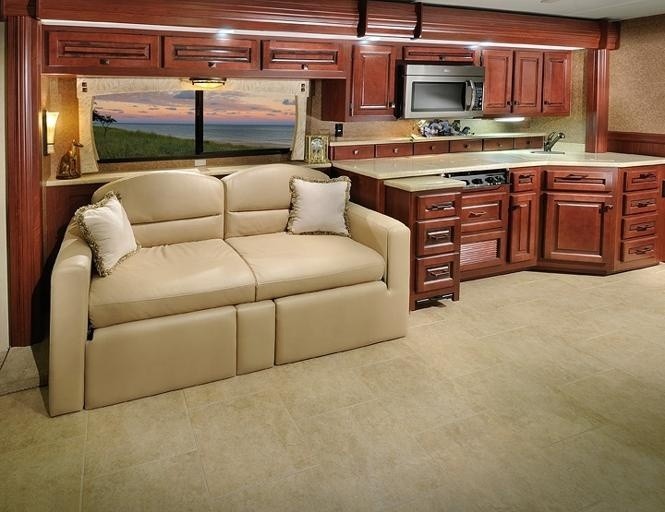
[48,163,411,418]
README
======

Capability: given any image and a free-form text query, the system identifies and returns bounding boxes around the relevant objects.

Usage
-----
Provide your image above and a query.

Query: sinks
[529,150,565,154]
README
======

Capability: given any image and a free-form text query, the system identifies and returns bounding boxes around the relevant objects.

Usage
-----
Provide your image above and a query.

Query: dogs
[59,136,83,177]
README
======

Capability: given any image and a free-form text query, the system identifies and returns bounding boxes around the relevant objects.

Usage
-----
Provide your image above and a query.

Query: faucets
[543,130,565,151]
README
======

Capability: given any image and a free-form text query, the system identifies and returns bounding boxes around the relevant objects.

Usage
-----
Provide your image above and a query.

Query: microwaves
[393,58,487,120]
